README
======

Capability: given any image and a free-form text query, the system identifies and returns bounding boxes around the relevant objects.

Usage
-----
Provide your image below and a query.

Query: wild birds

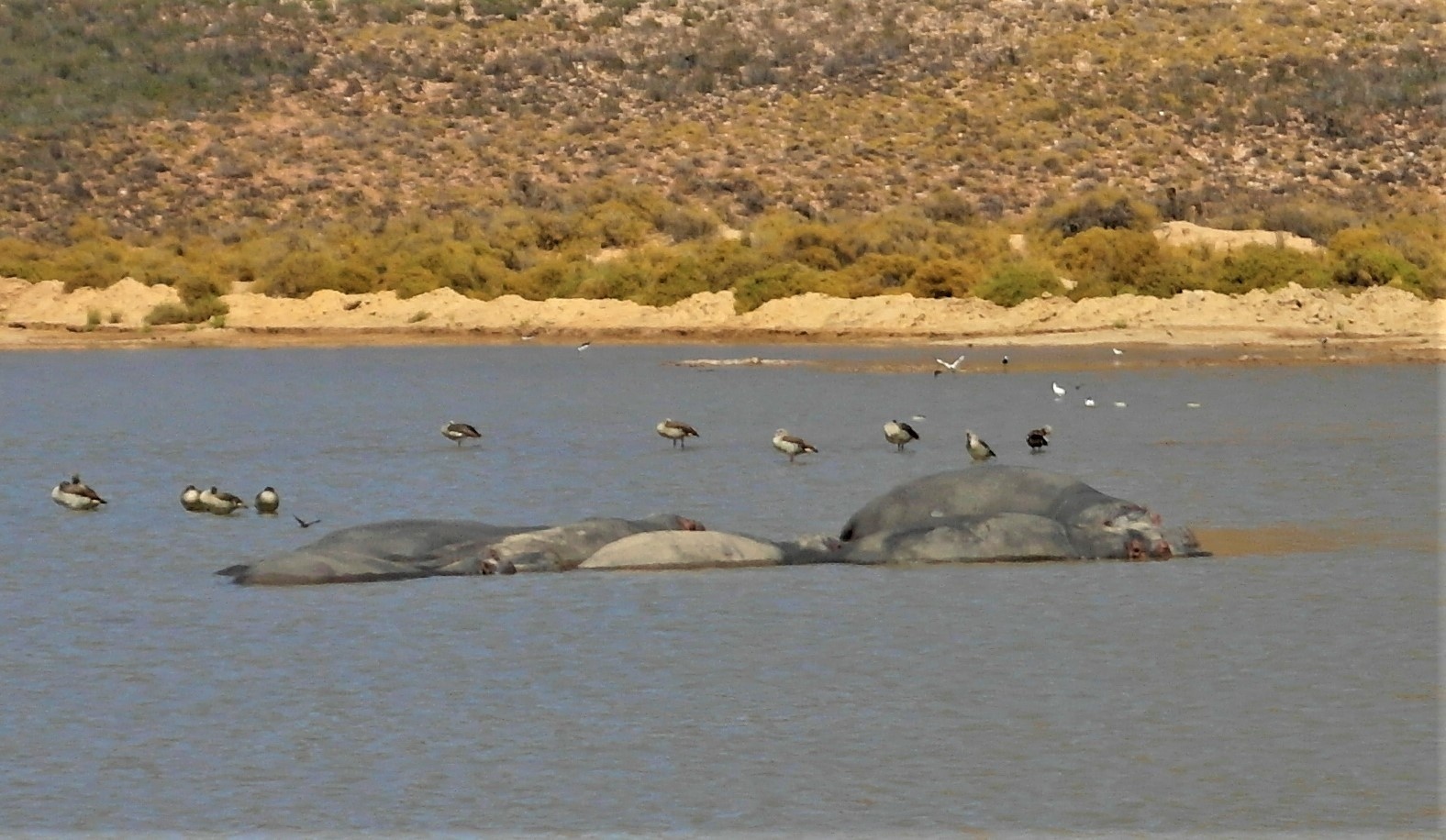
[578,341,591,351]
[180,484,242,508]
[656,418,700,446]
[51,473,108,509]
[1187,401,1201,408]
[255,486,280,511]
[935,355,965,371]
[1085,396,1095,407]
[1051,381,1066,396]
[1112,348,1124,355]
[1113,400,1128,407]
[964,428,996,459]
[1002,356,1009,364]
[772,428,818,461]
[440,418,482,447]
[883,420,919,450]
[293,514,321,527]
[1025,425,1052,449]
